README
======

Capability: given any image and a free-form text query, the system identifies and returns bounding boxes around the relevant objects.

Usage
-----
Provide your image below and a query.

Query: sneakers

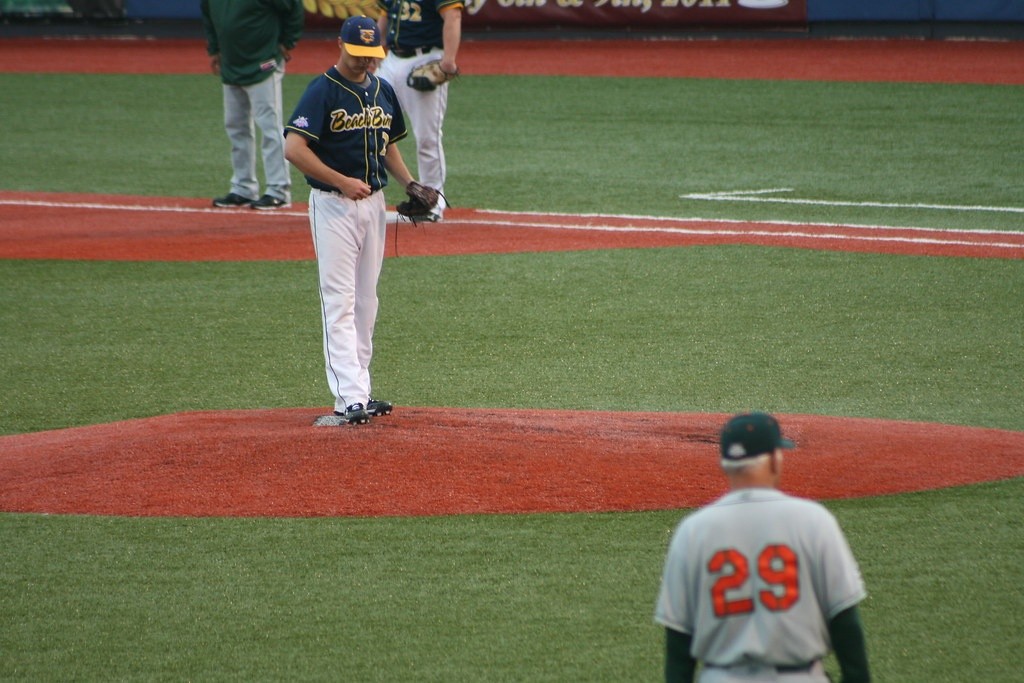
[213,192,255,208]
[334,400,393,415]
[250,194,290,211]
[345,403,369,425]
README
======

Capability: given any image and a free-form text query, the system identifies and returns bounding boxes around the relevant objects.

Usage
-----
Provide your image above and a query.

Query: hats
[341,14,386,59]
[720,414,796,460]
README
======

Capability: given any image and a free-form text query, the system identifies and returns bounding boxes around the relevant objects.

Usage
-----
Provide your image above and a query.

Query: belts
[389,45,436,58]
[776,662,814,673]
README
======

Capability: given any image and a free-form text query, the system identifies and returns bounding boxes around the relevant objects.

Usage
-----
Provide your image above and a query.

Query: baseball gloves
[395,177,446,222]
[407,59,460,91]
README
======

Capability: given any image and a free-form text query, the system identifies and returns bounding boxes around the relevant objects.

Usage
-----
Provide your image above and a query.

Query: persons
[200,0,304,210]
[284,16,421,423]
[373,0,463,221]
[655,414,869,683]
